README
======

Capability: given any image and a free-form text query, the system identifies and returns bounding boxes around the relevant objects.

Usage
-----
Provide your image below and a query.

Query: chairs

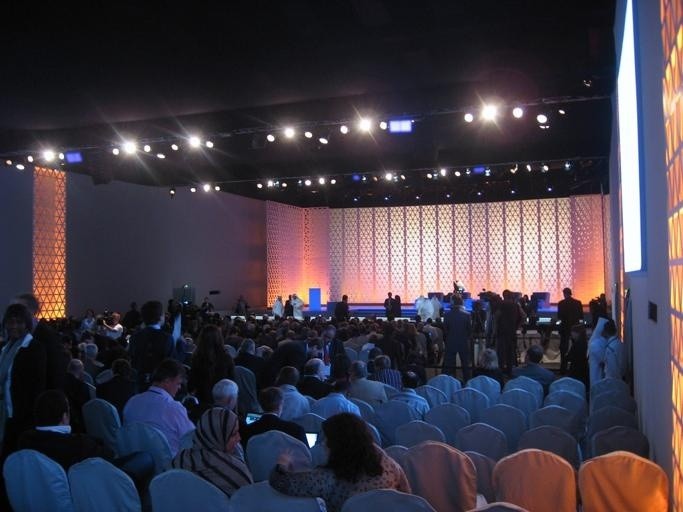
[293,413,327,432]
[311,445,333,466]
[116,422,174,476]
[375,400,415,445]
[67,458,140,512]
[2,450,72,512]
[480,403,529,453]
[366,423,383,448]
[81,398,119,449]
[543,389,587,434]
[177,427,244,462]
[527,404,580,443]
[358,349,369,361]
[345,347,357,362]
[148,470,228,512]
[383,384,398,400]
[395,420,446,449]
[590,391,637,413]
[425,403,472,448]
[465,502,528,512]
[502,376,542,408]
[428,374,461,404]
[591,377,630,398]
[466,375,500,404]
[497,388,537,430]
[583,406,636,457]
[349,397,374,423]
[397,441,477,512]
[245,430,311,481]
[576,451,670,512]
[491,447,578,512]
[228,480,327,512]
[464,451,497,504]
[549,378,586,398]
[455,423,507,458]
[341,489,437,512]
[519,426,579,469]
[417,384,446,406]
[452,388,489,424]
[383,445,409,469]
[586,427,650,461]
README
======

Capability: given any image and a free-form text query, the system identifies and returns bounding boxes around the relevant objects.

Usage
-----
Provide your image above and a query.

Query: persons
[6,293,53,390]
[589,317,608,384]
[563,325,591,393]
[166,407,253,498]
[557,288,585,375]
[123,302,141,327]
[376,324,404,362]
[590,298,599,323]
[335,295,349,319]
[476,348,504,393]
[524,295,529,303]
[0,304,48,512]
[168,298,177,327]
[318,324,345,367]
[275,366,310,419]
[82,343,104,379]
[456,279,465,293]
[382,314,399,337]
[241,387,310,452]
[348,360,388,407]
[494,290,515,370]
[340,314,383,349]
[273,336,325,367]
[510,292,526,366]
[272,295,287,317]
[76,434,153,511]
[65,359,91,399]
[444,294,473,388]
[192,378,245,428]
[234,338,270,391]
[416,295,425,310]
[396,315,443,370]
[233,313,318,344]
[527,295,539,325]
[598,294,608,319]
[431,294,442,321]
[93,324,102,334]
[270,413,413,512]
[204,309,210,315]
[183,331,196,353]
[102,311,123,340]
[453,281,459,294]
[187,325,235,405]
[39,315,80,335]
[599,321,627,379]
[283,294,293,317]
[311,380,361,420]
[123,356,196,460]
[96,358,138,424]
[390,370,430,415]
[15,389,78,463]
[127,300,176,391]
[384,292,398,321]
[181,306,203,325]
[470,301,487,366]
[417,297,435,320]
[201,296,215,314]
[184,299,189,305]
[511,345,554,396]
[189,298,193,305]
[367,354,403,392]
[80,309,97,330]
[233,292,252,314]
[204,313,232,324]
[292,294,305,320]
[58,335,72,351]
[394,295,401,318]
[105,341,127,355]
[478,288,487,301]
[80,330,95,343]
[174,296,184,312]
[296,358,335,400]
